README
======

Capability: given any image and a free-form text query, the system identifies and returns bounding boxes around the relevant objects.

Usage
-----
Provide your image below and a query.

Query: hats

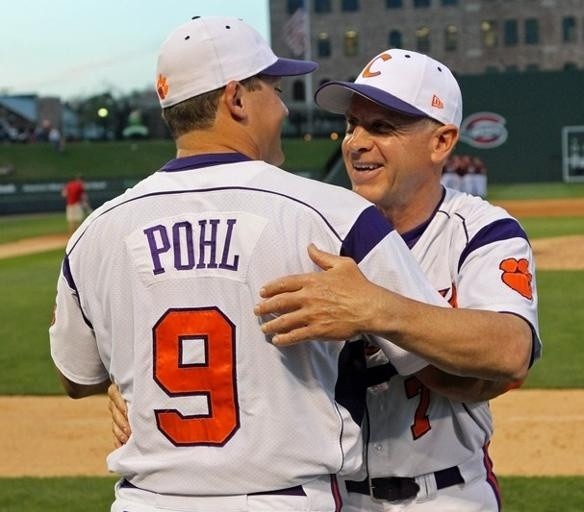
[154,13,319,109]
[311,46,466,134]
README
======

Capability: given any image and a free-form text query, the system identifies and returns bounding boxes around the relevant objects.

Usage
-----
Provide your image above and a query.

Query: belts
[341,465,465,504]
[120,477,307,497]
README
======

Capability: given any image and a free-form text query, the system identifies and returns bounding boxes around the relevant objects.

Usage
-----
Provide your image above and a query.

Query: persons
[46,124,59,154]
[439,152,488,199]
[48,15,526,511]
[104,46,544,511]
[59,172,92,238]
